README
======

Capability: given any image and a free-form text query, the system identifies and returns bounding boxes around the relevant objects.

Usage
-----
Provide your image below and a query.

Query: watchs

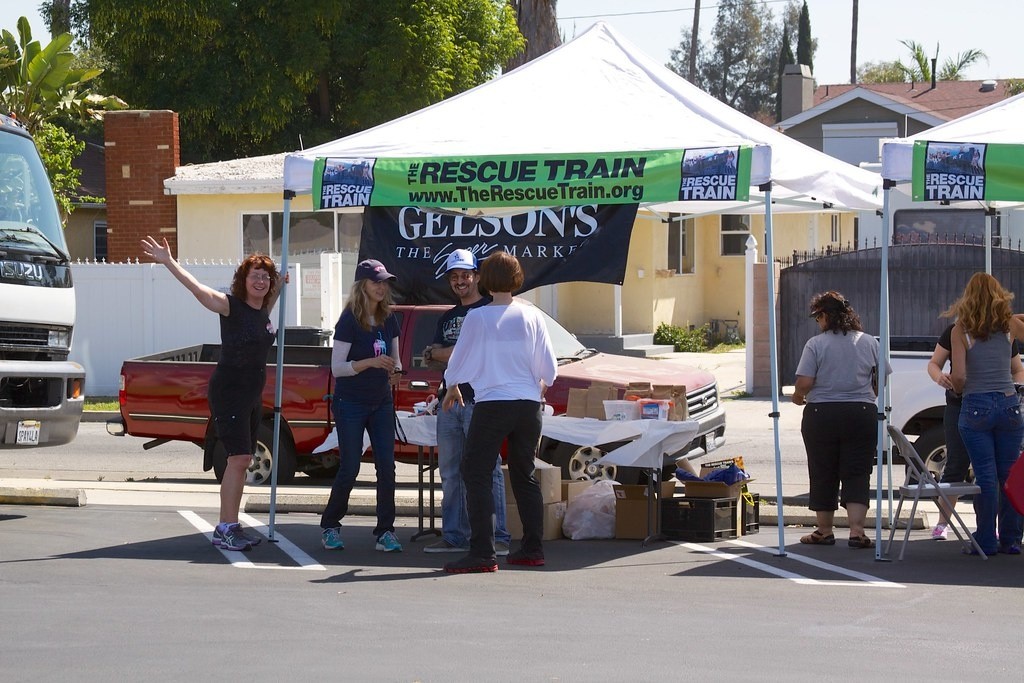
[425,348,432,360]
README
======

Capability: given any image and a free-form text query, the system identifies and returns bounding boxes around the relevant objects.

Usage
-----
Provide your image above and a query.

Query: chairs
[885,425,988,562]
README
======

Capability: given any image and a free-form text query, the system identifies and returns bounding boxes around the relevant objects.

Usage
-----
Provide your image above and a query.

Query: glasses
[244,273,281,285]
[815,313,820,323]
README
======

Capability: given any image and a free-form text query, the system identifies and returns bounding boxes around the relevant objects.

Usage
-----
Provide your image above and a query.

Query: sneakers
[932,524,951,541]
[374,530,403,554]
[318,525,345,549]
[212,524,266,548]
[219,523,259,551]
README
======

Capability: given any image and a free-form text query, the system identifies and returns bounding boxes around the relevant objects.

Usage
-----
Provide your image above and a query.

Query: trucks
[0,109,88,452]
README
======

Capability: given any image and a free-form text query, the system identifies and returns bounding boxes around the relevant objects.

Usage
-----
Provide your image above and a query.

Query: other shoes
[801,530,837,545]
[423,540,470,553]
[442,556,499,574]
[847,533,877,548]
[504,546,547,566]
[998,544,1021,555]
[494,540,511,557]
[962,543,998,556]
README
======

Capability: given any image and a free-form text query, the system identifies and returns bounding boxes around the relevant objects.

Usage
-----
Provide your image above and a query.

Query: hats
[443,249,483,273]
[354,259,401,283]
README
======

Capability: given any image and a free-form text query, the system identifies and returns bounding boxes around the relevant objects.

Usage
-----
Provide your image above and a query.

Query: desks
[312,407,699,549]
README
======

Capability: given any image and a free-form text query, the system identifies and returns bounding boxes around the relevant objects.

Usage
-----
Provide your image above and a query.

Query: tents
[875,92,1024,562]
[267,20,911,557]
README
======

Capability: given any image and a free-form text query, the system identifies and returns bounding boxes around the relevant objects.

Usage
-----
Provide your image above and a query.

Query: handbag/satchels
[564,479,624,541]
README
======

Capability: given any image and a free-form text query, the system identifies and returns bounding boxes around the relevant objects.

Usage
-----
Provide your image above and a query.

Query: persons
[422,249,512,555]
[927,323,1024,540]
[442,251,558,573]
[320,259,403,551]
[792,291,893,548]
[950,272,1024,555]
[140,236,290,551]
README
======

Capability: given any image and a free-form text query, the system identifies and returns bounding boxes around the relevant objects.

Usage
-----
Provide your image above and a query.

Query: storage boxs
[500,466,562,504]
[700,457,749,493]
[602,398,641,420]
[636,398,672,421]
[659,465,756,498]
[560,480,616,540]
[505,502,567,541]
[612,482,676,539]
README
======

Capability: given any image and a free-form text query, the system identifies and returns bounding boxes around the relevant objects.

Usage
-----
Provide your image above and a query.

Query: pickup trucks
[873,349,1024,500]
[106,297,728,487]
